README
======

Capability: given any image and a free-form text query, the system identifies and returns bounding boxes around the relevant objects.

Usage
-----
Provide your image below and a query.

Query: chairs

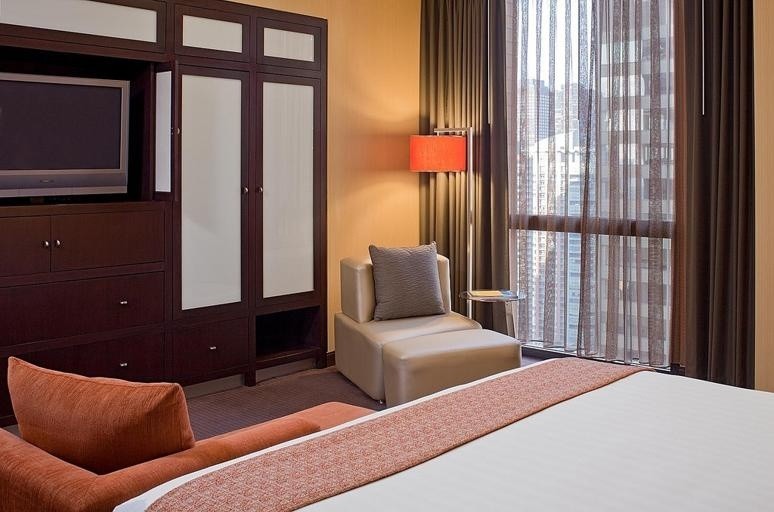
[333,253,483,405]
[1,418,320,511]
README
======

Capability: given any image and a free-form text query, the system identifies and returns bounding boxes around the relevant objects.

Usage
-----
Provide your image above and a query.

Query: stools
[382,328,522,409]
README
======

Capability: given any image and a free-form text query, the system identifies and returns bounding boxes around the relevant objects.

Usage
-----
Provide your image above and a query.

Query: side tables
[460,289,530,345]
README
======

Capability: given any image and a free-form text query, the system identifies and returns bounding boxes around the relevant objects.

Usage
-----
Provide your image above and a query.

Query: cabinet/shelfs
[1,199,173,428]
[132,1,328,401]
[1,1,169,63]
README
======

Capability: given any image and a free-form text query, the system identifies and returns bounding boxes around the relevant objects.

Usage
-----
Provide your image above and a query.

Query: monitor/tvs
[0,73,131,198]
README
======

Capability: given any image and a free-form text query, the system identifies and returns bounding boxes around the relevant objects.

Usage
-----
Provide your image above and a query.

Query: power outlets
[409,127,474,322]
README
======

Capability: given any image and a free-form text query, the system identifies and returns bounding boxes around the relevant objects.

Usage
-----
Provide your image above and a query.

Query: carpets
[199,401,378,442]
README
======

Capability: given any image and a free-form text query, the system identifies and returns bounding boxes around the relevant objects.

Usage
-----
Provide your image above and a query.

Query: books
[468,290,503,297]
[466,290,518,299]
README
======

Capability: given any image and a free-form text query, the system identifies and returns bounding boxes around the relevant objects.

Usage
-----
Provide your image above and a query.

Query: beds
[109,356,774,512]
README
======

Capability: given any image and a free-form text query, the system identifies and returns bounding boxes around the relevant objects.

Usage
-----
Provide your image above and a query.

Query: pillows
[6,354,197,474]
[369,241,446,320]
